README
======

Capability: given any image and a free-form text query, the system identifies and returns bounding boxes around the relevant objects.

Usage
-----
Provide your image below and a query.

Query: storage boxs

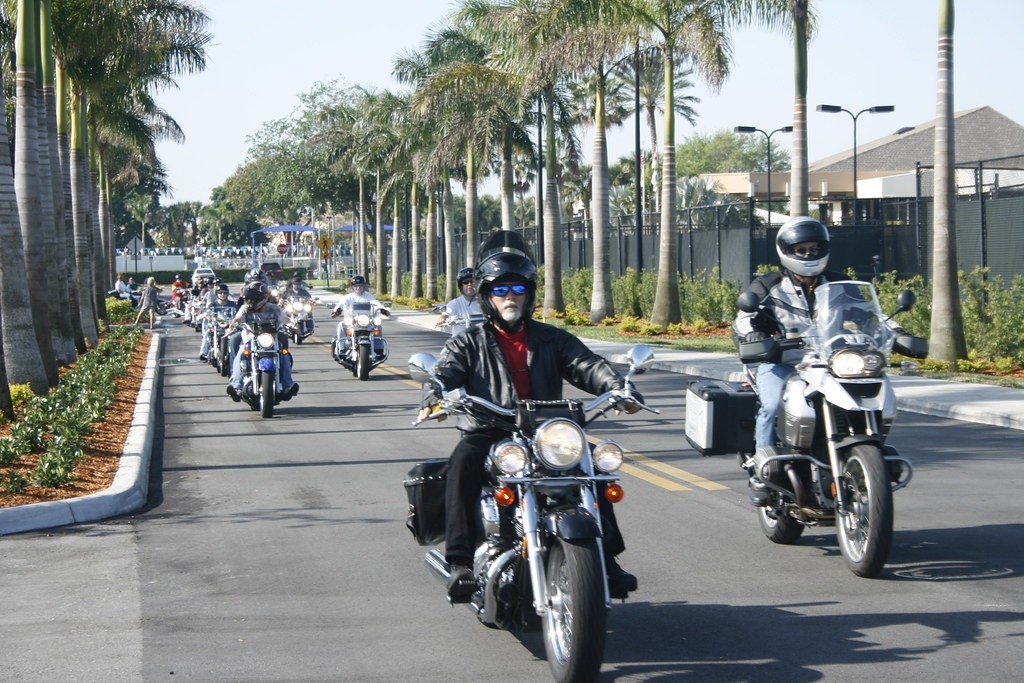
[685,380,759,456]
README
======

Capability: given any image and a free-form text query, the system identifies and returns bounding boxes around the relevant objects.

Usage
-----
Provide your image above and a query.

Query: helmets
[243,281,269,313]
[243,269,303,284]
[472,252,537,321]
[175,273,231,296]
[458,268,477,292]
[351,276,366,286]
[776,216,830,276]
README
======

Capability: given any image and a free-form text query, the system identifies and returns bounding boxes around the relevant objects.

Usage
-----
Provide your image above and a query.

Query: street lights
[733,124,793,274]
[124,246,129,277]
[815,102,896,230]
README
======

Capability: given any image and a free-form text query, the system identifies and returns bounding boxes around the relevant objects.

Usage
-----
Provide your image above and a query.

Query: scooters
[433,300,490,335]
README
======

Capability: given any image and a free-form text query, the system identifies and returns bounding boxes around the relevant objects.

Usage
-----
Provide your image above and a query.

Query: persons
[172,274,189,309]
[114,275,126,293]
[127,277,138,294]
[183,268,299,401]
[729,217,928,496]
[331,277,392,354]
[413,230,642,603]
[272,273,318,338]
[136,277,162,328]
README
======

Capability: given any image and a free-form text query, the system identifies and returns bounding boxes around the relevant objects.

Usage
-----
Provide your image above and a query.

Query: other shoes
[281,382,299,401]
[199,355,208,365]
[446,564,477,605]
[750,477,772,507]
[227,385,240,402]
[175,313,198,330]
[608,554,638,593]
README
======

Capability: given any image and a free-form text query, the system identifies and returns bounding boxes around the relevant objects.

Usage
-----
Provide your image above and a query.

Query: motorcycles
[223,319,294,419]
[733,280,932,576]
[155,291,239,378]
[324,300,393,383]
[404,344,662,682]
[272,291,320,347]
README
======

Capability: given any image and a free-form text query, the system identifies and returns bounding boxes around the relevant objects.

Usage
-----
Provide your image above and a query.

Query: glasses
[460,279,474,284]
[793,247,818,254]
[486,283,528,296]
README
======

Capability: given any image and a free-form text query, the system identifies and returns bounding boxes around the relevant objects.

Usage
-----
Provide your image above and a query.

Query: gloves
[746,330,768,344]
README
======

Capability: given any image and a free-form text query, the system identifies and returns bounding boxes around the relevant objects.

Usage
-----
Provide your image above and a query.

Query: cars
[191,268,216,286]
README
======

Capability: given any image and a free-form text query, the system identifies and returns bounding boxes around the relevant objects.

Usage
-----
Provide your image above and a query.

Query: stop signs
[278,244,288,254]
